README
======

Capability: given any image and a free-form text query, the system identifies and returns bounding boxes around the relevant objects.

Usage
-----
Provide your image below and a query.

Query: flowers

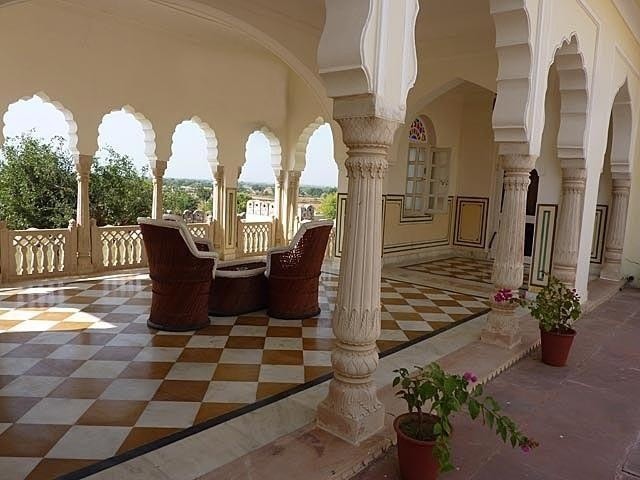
[494,270,581,333]
[392,361,540,474]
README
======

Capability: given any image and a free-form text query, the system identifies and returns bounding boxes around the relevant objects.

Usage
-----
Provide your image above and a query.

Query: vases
[393,412,456,480]
[539,322,577,367]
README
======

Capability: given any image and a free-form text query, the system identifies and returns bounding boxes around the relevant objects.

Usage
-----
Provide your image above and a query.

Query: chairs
[136,216,221,331]
[264,221,334,320]
